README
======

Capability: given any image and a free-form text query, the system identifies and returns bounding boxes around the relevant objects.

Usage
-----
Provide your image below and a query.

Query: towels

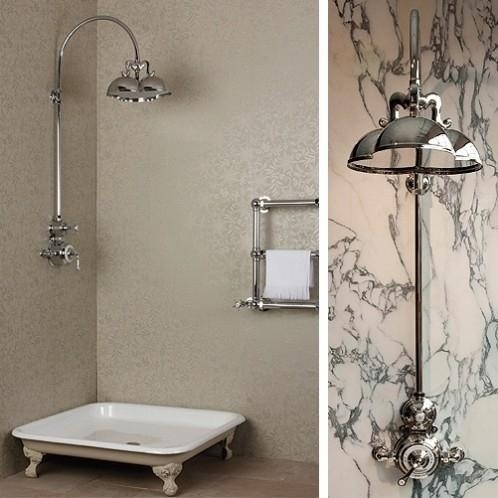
[264,250,314,301]
